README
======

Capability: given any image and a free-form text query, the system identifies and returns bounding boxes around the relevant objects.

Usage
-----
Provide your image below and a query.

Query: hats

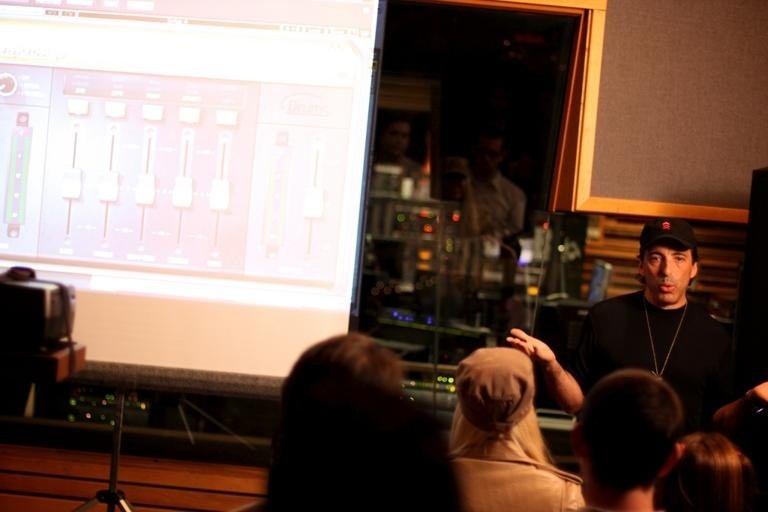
[639,216,695,251]
[456,347,534,433]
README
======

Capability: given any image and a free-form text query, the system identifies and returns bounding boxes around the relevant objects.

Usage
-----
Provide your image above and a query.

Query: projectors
[0,264,77,338]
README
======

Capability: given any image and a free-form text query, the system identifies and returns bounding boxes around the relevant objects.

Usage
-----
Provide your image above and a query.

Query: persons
[506,218,768,431]
[445,125,528,239]
[373,118,421,167]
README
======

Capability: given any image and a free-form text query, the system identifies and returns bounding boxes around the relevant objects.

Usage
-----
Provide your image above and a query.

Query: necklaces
[644,295,688,377]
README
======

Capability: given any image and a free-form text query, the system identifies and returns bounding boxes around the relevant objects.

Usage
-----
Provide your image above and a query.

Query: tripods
[68,394,133,512]
